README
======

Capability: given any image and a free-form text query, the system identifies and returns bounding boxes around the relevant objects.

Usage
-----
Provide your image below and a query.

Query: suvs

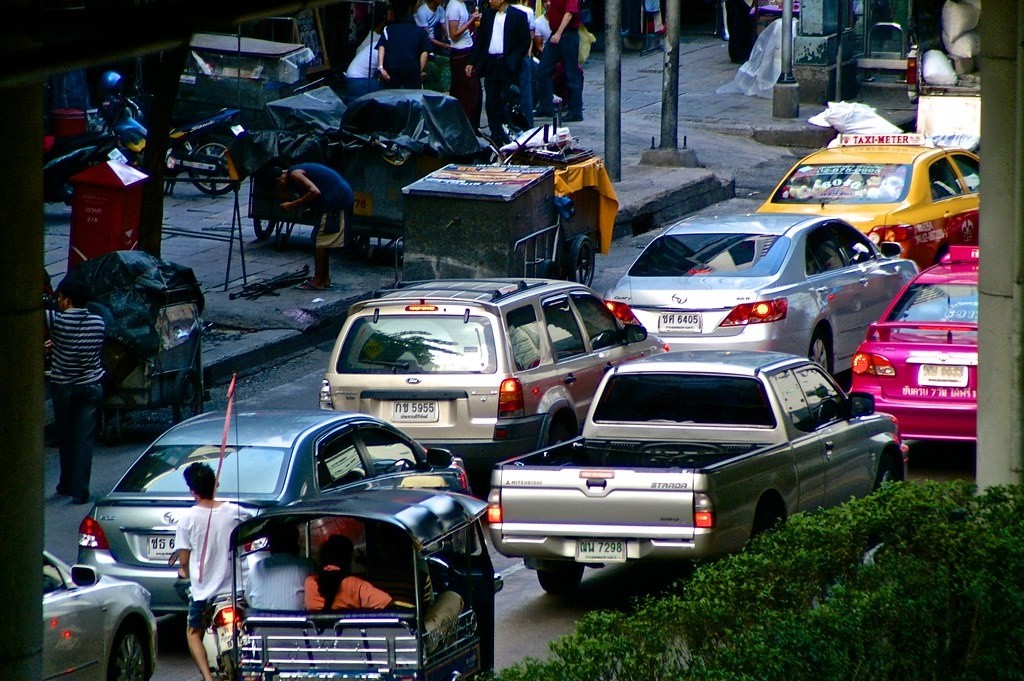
[317,275,672,492]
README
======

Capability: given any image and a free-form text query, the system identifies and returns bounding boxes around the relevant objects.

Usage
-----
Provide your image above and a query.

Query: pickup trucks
[488,346,909,599]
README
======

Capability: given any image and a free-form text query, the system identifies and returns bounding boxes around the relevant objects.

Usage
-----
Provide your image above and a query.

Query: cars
[602,212,922,380]
[754,132,982,273]
[842,245,981,450]
[40,550,160,681]
[75,408,474,631]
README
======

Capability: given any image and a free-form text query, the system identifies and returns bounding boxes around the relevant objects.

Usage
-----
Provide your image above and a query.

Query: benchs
[242,606,430,669]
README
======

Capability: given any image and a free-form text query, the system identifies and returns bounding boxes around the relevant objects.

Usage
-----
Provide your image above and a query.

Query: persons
[245,523,315,610]
[45,279,105,504]
[268,163,354,290]
[174,463,253,681]
[344,0,584,146]
[304,534,463,654]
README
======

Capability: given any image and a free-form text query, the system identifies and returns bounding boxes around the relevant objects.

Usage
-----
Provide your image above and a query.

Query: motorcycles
[59,74,247,199]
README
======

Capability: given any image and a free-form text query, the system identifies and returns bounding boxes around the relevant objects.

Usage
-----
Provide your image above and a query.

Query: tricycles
[388,122,617,290]
[225,478,505,681]
[245,83,492,267]
[56,250,209,435]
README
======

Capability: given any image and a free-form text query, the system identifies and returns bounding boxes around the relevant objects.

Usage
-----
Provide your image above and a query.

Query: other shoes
[56,482,74,495]
[561,112,583,122]
[533,105,556,118]
[65,497,88,507]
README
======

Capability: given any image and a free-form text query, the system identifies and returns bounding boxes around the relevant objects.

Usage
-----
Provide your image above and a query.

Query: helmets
[98,69,122,92]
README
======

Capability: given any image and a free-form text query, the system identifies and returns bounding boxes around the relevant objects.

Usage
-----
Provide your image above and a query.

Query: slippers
[294,277,334,291]
